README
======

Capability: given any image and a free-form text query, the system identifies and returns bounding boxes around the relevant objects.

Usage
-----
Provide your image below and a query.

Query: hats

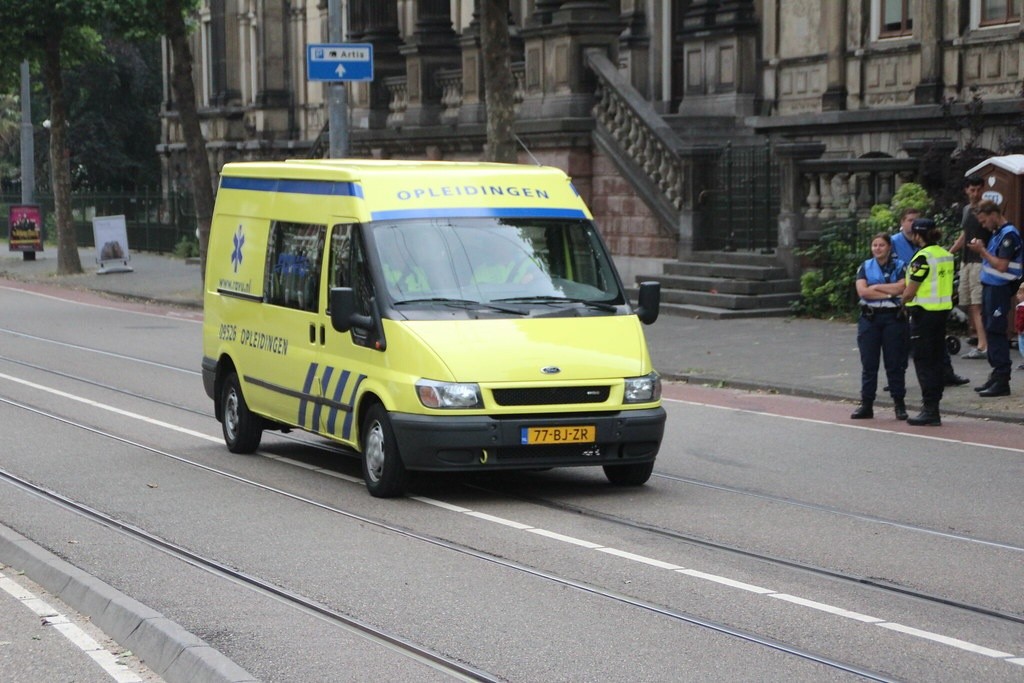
[912,218,934,230]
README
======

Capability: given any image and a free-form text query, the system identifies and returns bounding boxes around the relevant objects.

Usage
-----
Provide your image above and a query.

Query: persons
[467,228,542,292]
[375,237,431,298]
[850,175,1024,425]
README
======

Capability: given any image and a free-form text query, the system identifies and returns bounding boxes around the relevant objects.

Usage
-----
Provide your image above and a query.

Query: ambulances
[198,157,667,501]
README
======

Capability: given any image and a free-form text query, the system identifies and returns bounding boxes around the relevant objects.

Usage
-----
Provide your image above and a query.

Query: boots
[892,396,908,420]
[850,398,874,418]
[907,396,941,425]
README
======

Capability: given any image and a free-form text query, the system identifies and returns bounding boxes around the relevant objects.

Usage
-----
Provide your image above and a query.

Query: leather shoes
[975,380,995,392]
[979,381,1010,396]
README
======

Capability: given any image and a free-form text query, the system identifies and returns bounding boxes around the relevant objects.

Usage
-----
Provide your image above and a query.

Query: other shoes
[967,336,979,345]
[1009,339,1019,350]
[1017,364,1024,369]
[960,347,987,359]
[943,374,970,385]
